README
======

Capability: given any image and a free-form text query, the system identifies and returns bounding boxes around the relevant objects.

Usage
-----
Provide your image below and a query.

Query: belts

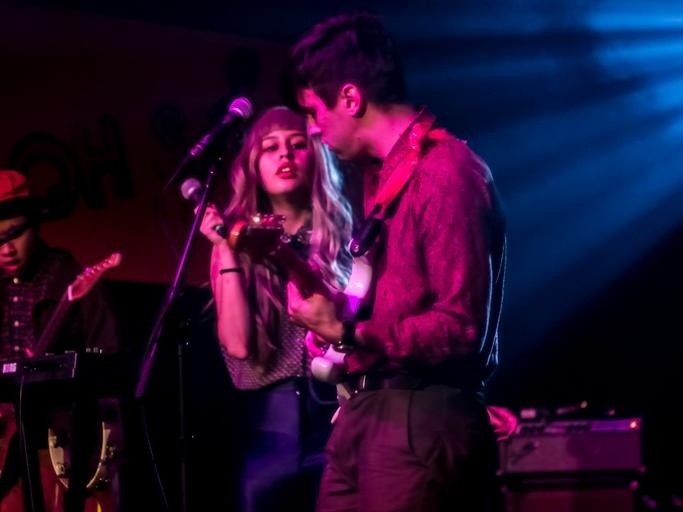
[351,372,486,392]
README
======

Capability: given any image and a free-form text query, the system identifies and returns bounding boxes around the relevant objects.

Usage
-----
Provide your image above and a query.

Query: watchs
[331,321,358,353]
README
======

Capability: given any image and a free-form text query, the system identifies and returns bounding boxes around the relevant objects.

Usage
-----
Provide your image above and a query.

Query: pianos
[0,348,129,404]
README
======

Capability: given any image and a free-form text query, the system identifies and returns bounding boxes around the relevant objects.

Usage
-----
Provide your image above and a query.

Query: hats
[0,168,35,220]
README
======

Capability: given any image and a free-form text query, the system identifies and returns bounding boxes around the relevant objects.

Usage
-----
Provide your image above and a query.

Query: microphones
[181,178,225,239]
[189,93,254,156]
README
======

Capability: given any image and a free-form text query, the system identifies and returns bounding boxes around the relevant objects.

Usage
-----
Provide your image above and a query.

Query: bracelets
[216,266,243,276]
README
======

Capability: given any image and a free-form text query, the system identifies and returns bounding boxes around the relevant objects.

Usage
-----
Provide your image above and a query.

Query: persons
[288,14,510,511]
[1,168,134,512]
[192,106,376,512]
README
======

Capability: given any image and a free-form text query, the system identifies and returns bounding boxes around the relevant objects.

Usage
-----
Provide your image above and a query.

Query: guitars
[24,251,124,359]
[228,214,378,385]
